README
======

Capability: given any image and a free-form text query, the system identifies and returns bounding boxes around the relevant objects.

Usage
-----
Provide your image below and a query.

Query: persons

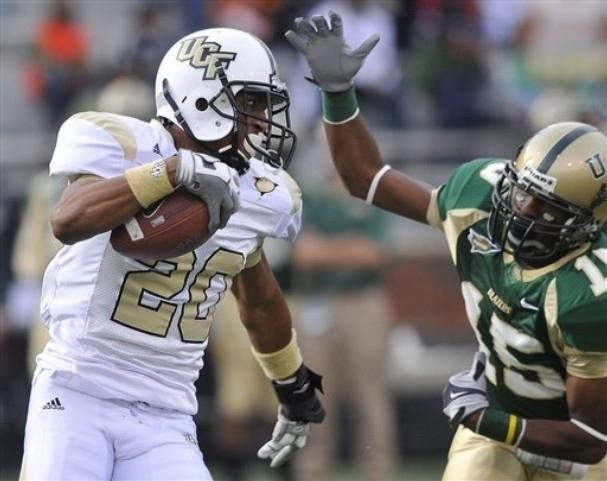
[0,77,397,481]
[286,12,606,480]
[19,27,325,480]
[0,1,606,130]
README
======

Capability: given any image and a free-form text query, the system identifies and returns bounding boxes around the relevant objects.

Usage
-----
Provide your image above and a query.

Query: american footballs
[109,187,225,260]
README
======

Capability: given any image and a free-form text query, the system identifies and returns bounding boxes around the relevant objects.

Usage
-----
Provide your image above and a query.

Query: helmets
[505,122,607,252]
[155,28,285,143]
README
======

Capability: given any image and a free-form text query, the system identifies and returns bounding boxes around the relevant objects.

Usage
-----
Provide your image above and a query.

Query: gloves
[176,146,241,232]
[442,351,490,431]
[257,405,309,468]
[285,10,379,94]
